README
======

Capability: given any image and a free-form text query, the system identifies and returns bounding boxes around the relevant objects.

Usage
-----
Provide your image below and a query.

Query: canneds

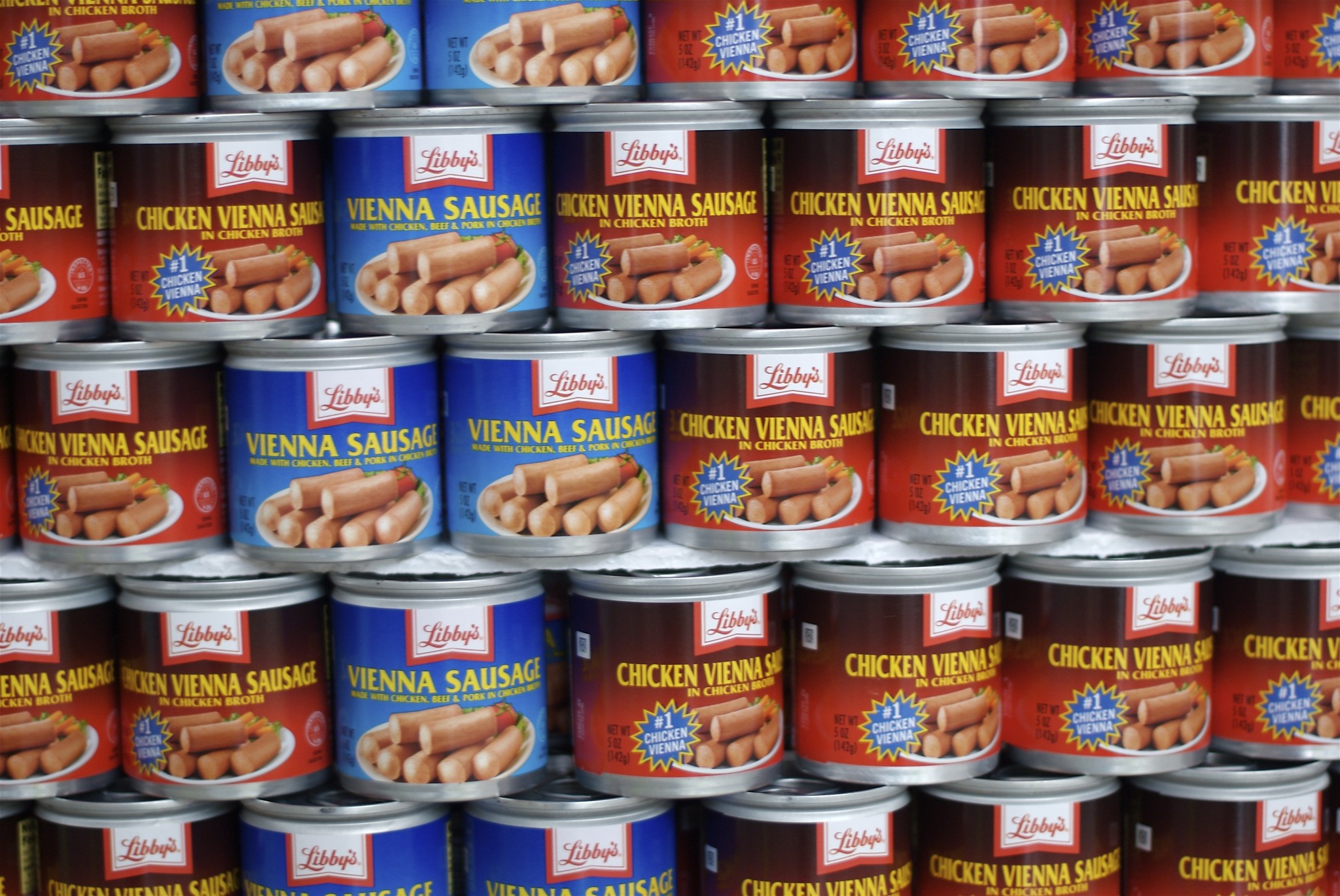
[1,0,1337,896]
